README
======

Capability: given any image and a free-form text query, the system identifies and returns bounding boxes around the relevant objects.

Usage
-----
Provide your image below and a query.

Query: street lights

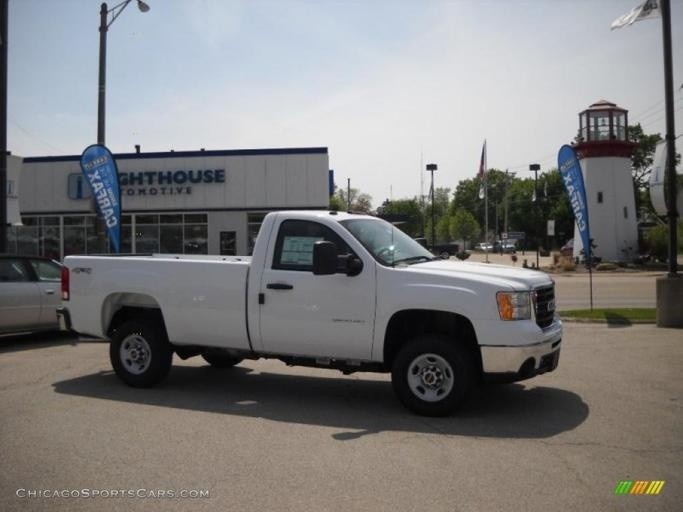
[529,163,542,269]
[426,163,438,255]
[95,0,151,256]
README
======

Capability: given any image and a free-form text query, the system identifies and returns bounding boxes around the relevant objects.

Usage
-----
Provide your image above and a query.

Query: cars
[475,239,520,254]
[0,252,62,338]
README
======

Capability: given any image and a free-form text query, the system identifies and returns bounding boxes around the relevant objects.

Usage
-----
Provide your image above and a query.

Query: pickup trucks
[56,206,564,418]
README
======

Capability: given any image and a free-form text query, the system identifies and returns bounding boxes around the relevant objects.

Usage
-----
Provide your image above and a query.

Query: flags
[476,138,489,200]
